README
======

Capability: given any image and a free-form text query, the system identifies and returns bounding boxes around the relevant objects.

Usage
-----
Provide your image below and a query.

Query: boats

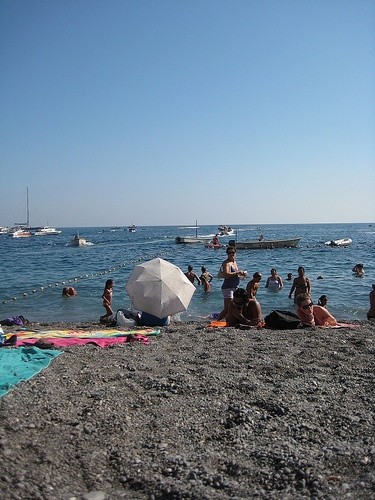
[174,220,211,244]
[204,242,224,249]
[108,224,140,232]
[323,238,353,246]
[225,238,302,250]
[0,186,63,239]
[217,226,235,236]
[71,230,87,245]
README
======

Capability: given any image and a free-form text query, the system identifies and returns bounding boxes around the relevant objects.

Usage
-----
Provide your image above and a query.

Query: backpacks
[263,309,302,330]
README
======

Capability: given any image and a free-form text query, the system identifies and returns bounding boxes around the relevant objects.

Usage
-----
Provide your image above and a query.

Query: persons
[317,295,328,309]
[0,315,29,326]
[62,286,76,296]
[213,234,219,245]
[102,279,114,315]
[295,294,337,327]
[219,246,247,319]
[367,284,375,319]
[329,240,337,246]
[199,275,210,292]
[200,266,214,284]
[246,272,262,298]
[286,273,292,280]
[118,311,170,326]
[288,266,311,304]
[184,265,201,286]
[352,263,364,275]
[265,268,283,288]
[227,288,262,326]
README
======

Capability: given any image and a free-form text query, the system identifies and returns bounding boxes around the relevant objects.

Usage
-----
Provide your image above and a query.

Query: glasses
[227,251,236,254]
[232,298,245,306]
[301,300,314,310]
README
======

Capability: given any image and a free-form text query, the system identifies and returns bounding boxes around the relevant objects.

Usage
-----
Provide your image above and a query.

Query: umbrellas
[125,257,196,318]
